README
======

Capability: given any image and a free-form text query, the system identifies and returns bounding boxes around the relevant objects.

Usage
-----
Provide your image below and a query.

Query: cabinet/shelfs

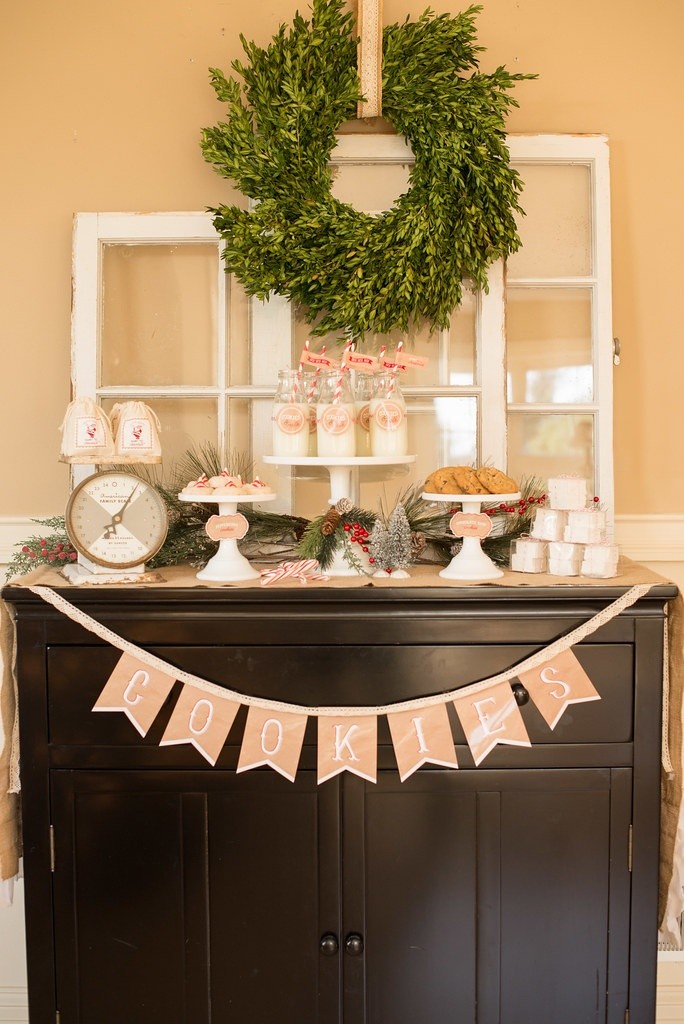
[0,556,679,1024]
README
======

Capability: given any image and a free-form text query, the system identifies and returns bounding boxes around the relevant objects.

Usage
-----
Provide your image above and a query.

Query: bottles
[370,375,406,457]
[316,371,355,457]
[271,370,310,457]
[355,373,373,457]
[302,371,318,457]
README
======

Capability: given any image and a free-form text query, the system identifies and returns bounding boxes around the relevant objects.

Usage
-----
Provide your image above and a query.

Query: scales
[56,453,172,583]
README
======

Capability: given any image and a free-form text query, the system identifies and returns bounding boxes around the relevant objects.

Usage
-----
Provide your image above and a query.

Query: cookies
[423,466,518,495]
